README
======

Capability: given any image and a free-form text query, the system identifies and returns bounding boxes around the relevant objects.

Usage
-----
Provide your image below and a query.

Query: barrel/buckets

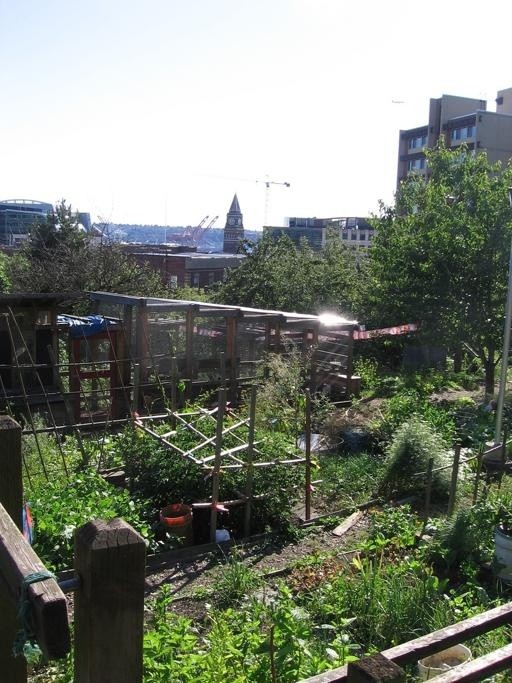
[495,526,511,580]
[417,643,473,682]
[159,504,194,547]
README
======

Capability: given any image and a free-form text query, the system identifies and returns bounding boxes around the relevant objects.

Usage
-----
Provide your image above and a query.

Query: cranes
[254,178,291,226]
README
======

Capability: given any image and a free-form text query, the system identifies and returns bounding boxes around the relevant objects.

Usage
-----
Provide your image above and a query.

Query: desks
[331,375,362,408]
